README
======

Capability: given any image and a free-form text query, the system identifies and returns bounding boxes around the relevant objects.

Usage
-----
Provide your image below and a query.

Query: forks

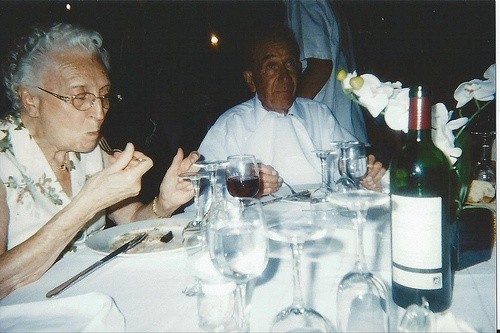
[98,137,147,162]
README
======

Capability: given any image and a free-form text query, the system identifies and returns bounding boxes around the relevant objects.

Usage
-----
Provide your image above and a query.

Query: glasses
[38,84,115,112]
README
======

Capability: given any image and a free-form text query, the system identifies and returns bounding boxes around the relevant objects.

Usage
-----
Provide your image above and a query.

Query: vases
[448,130,472,219]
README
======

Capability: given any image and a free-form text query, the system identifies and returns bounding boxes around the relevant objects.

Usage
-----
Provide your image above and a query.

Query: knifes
[46,232,148,298]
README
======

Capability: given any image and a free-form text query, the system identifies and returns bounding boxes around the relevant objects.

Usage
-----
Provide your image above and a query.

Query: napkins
[0,293,125,333]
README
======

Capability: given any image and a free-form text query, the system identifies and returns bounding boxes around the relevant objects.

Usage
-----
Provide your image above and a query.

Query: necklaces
[51,159,68,171]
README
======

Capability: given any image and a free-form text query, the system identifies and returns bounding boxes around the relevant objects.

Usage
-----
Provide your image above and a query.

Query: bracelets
[151,196,171,218]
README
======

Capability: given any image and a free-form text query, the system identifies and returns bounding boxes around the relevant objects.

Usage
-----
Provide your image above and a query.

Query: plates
[280,189,329,204]
[86,217,205,256]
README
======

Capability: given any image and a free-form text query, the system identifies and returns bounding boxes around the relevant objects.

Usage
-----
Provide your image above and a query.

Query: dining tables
[0,176,496,333]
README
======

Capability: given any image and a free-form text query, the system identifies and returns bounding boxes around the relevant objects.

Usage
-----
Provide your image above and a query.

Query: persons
[0,18,202,304]
[283,0,376,157]
[190,24,385,200]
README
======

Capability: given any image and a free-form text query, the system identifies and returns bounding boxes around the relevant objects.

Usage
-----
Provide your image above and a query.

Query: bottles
[390,85,453,314]
[176,159,226,256]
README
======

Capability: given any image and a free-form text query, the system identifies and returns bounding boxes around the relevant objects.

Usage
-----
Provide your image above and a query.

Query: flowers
[337,59,496,173]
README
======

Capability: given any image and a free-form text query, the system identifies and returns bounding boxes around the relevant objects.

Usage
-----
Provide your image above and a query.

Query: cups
[226,153,260,196]
[339,141,369,179]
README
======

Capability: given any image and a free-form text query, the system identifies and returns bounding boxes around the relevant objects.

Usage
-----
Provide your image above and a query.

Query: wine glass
[262,218,332,333]
[472,130,496,187]
[325,190,391,333]
[206,196,270,333]
[310,140,357,218]
